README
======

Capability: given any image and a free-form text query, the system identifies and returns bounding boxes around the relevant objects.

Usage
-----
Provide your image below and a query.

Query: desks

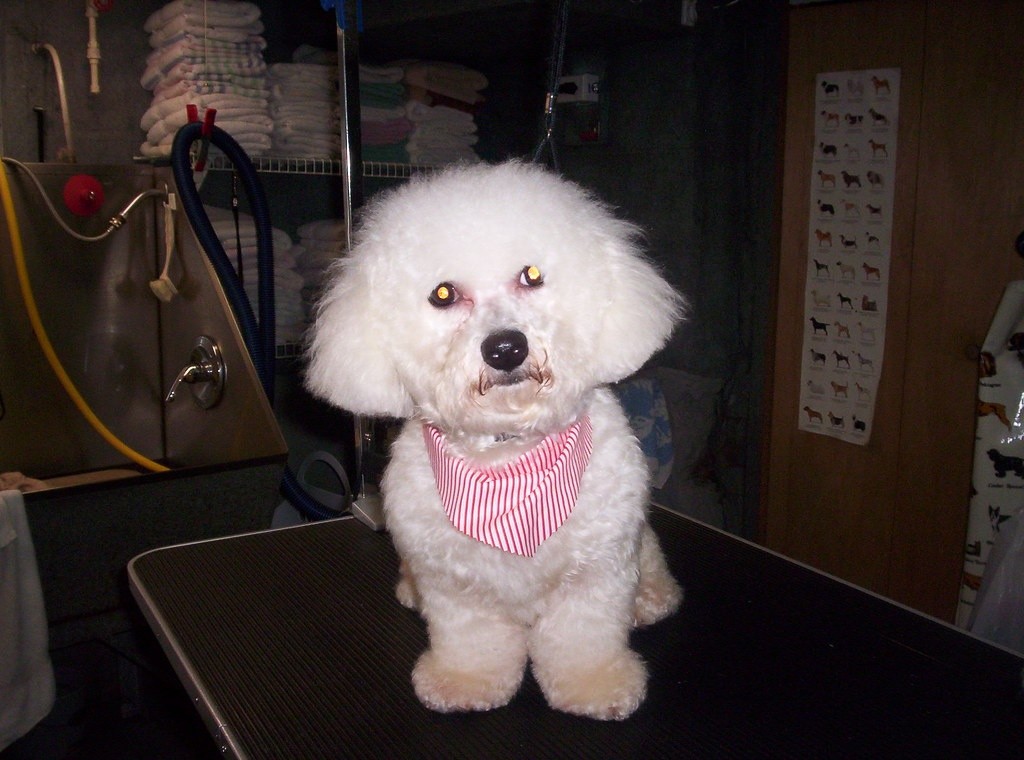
[127,500,1024,760]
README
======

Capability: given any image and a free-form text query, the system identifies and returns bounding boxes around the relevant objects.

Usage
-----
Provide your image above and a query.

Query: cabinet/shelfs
[125,152,434,357]
[758,0,1024,628]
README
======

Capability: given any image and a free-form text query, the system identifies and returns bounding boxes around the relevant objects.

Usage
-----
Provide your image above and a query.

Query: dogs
[303,158,687,723]
